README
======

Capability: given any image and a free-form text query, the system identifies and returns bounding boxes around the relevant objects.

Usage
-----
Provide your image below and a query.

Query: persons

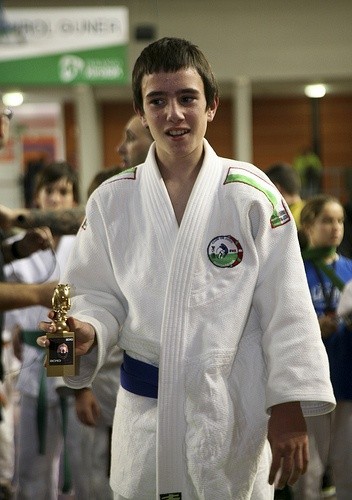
[53,163,123,500]
[289,192,352,500]
[263,161,308,251]
[0,115,154,236]
[2,161,76,500]
[36,37,336,500]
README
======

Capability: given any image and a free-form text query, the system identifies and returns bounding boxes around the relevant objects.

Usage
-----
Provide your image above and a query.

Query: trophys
[45,283,76,377]
